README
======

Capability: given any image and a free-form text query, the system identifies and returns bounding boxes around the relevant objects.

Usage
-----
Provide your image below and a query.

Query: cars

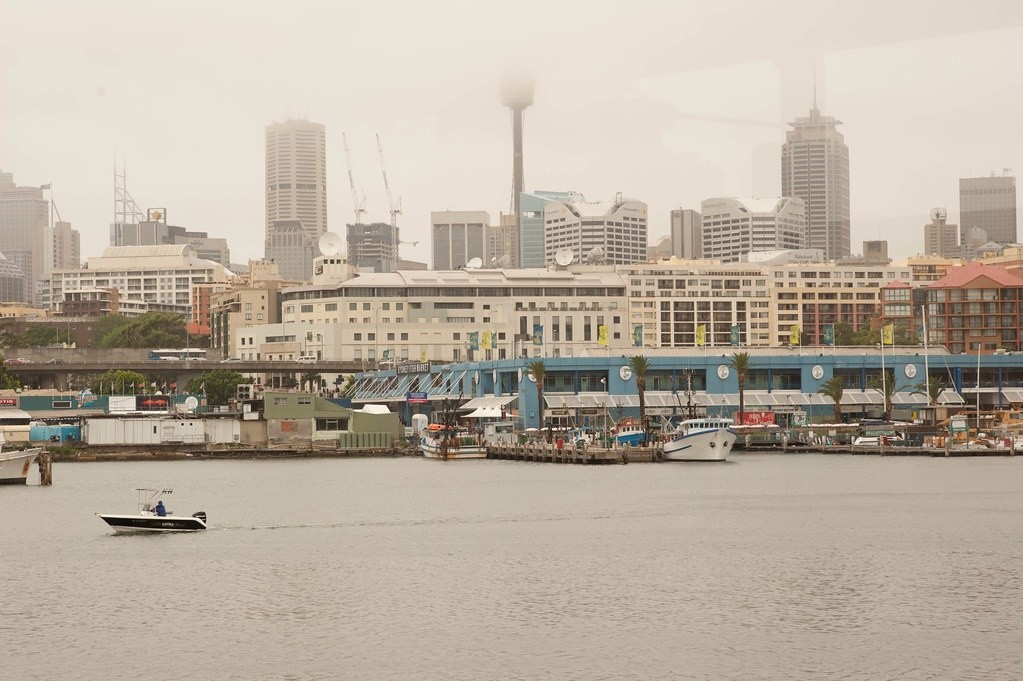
[219,358,240,364]
[2,359,22,366]
[16,358,33,365]
[45,358,64,365]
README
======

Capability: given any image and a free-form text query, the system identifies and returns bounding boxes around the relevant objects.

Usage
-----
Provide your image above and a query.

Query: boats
[94,488,207,533]
[419,390,1023,466]
[0,447,41,485]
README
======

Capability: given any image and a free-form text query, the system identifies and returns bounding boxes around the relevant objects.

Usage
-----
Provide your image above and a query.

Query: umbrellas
[152,400,169,411]
[141,400,156,411]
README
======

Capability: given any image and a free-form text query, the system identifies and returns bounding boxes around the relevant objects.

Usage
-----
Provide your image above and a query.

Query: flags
[533,324,544,346]
[598,326,608,346]
[823,325,833,344]
[789,325,800,345]
[696,324,705,344]
[882,324,892,345]
[634,325,643,347]
[381,329,499,365]
[100,377,178,395]
[730,325,739,344]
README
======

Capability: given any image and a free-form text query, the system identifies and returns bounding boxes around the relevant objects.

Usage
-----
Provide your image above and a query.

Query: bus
[148,348,207,362]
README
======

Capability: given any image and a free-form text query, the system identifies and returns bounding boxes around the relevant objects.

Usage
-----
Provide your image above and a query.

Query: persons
[151,501,167,517]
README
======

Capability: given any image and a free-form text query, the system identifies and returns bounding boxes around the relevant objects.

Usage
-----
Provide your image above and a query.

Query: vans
[295,356,317,365]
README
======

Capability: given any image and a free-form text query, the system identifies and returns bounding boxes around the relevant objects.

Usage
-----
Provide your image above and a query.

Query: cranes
[375,132,403,273]
[342,132,368,270]
